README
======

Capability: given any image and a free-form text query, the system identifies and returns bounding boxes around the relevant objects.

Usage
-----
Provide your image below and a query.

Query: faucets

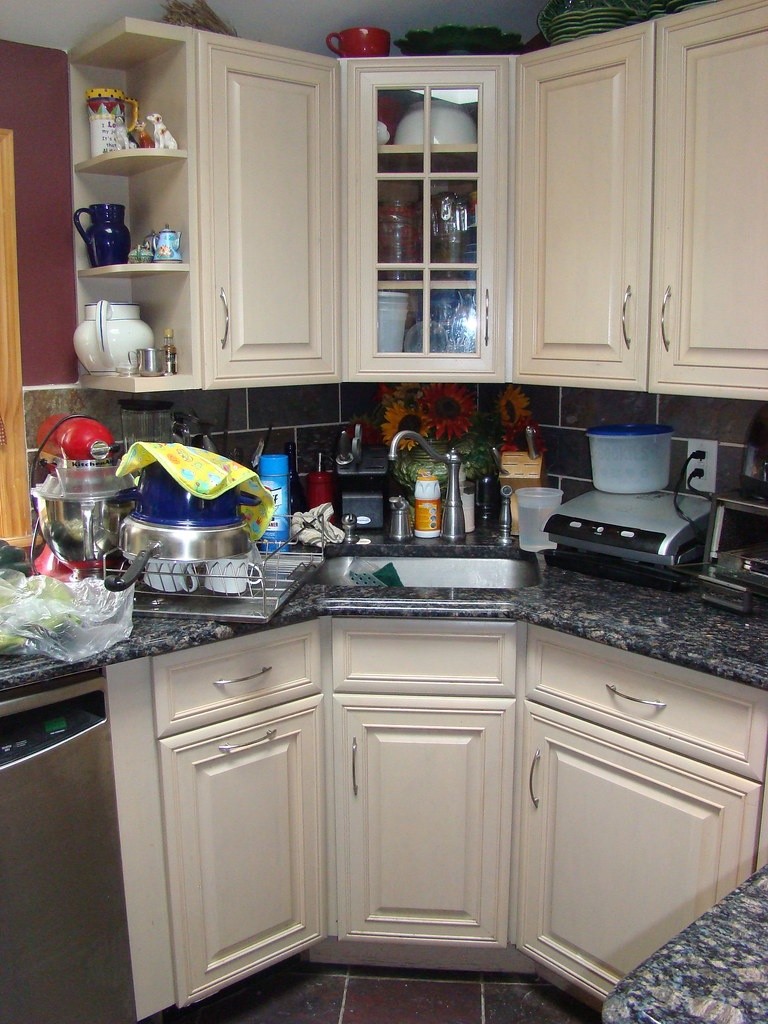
[388,429,467,542]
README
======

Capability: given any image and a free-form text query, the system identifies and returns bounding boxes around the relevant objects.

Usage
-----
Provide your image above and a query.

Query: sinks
[306,554,535,589]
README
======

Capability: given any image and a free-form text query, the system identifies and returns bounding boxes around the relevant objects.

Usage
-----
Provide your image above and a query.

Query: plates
[535,0,718,46]
[394,25,526,56]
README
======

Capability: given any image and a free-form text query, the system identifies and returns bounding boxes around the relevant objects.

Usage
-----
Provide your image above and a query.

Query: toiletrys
[413,468,441,539]
[258,453,290,552]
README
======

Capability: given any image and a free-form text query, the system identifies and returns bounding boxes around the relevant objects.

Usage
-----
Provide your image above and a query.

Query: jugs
[72,301,156,375]
[73,203,130,269]
[377,293,409,353]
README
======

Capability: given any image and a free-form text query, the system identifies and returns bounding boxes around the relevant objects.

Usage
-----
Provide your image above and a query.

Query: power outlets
[687,438,718,493]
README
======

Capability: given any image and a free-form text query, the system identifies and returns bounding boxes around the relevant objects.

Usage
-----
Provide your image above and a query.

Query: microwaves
[669,490,768,612]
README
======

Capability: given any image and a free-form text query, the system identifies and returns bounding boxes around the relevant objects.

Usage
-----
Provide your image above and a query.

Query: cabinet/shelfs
[343,55,509,384]
[126,16,340,397]
[151,616,328,1011]
[326,615,521,972]
[509,0,768,402]
[510,619,767,1011]
[66,19,204,392]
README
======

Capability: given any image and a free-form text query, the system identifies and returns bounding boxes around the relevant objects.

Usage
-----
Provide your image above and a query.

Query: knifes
[490,447,512,478]
[524,425,543,459]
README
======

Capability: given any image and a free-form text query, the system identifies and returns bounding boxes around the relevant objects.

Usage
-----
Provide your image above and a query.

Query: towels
[290,502,346,548]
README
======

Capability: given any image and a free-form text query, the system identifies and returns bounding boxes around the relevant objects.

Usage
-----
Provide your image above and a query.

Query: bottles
[259,441,332,552]
[162,327,178,375]
[456,461,476,532]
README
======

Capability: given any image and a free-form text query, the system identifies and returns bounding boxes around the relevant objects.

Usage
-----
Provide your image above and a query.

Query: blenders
[32,416,127,583]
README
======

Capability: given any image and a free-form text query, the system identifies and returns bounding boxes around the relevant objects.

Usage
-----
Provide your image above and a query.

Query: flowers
[350,382,548,478]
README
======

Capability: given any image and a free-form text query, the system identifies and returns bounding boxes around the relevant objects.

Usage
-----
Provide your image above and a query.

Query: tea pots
[129,222,183,262]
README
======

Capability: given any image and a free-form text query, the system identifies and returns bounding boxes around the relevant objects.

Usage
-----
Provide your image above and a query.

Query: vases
[389,439,471,505]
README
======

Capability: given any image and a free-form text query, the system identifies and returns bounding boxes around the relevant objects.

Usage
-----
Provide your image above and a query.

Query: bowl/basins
[586,423,674,494]
[103,461,259,594]
[28,473,135,573]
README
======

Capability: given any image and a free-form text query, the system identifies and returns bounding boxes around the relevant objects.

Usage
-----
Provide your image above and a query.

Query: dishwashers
[0,680,139,1024]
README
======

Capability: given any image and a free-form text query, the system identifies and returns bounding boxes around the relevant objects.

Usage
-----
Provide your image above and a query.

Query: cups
[128,347,167,377]
[516,487,563,554]
[325,27,391,58]
